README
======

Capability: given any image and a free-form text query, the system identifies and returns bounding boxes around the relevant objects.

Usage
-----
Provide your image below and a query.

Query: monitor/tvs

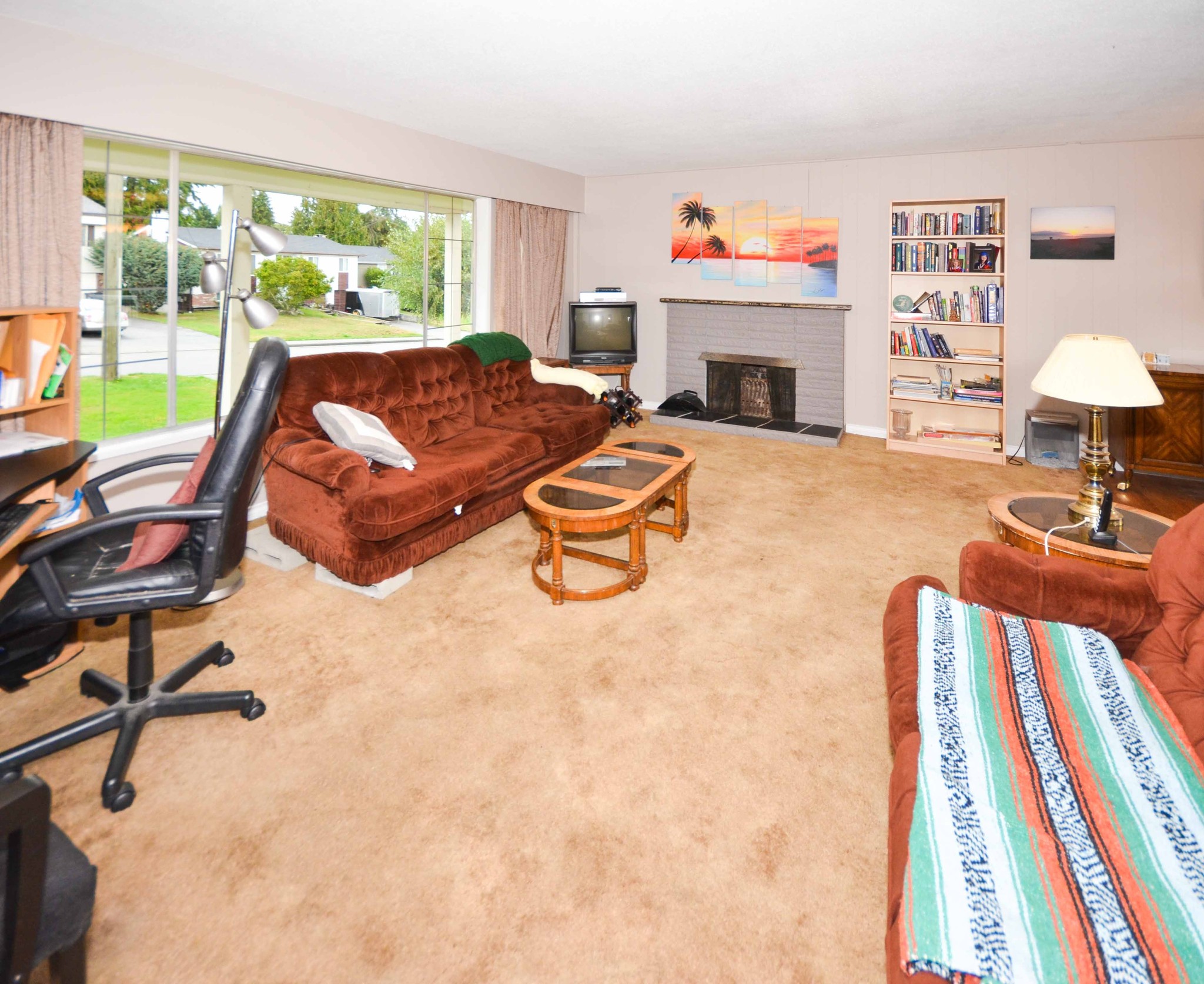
[568,302,638,366]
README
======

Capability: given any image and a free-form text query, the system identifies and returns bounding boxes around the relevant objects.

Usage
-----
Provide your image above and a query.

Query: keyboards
[0,503,41,547]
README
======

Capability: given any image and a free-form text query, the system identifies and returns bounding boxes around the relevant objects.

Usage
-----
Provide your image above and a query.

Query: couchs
[883,504,1204,984]
[262,332,611,585]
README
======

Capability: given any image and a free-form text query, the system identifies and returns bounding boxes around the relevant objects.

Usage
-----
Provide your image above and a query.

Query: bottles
[601,386,636,428]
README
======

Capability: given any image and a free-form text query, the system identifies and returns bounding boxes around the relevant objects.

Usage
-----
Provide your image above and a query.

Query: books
[952,379,1003,403]
[891,374,941,400]
[909,284,1004,324]
[892,241,975,273]
[891,323,1003,362]
[892,203,1001,236]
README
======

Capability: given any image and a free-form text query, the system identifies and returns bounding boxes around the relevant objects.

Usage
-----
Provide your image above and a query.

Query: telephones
[1087,488,1119,546]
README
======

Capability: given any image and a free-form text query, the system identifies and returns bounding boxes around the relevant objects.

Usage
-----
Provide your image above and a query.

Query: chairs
[0,771,95,984]
[0,336,289,813]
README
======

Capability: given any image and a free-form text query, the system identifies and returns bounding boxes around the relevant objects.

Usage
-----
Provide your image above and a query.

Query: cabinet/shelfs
[0,306,90,680]
[1107,364,1204,489]
[886,196,1008,466]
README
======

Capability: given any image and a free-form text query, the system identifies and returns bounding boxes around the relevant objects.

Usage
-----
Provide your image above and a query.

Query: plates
[893,295,914,313]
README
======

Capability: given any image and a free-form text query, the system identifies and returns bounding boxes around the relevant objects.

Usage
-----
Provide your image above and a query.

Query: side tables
[532,357,569,367]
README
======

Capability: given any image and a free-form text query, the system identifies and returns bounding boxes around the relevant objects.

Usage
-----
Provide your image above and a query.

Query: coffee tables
[522,439,696,605]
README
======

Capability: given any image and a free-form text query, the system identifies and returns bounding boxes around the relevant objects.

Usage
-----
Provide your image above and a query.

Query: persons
[974,252,991,269]
[948,259,963,273]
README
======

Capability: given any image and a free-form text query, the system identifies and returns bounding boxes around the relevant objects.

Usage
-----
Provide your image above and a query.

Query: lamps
[198,210,288,606]
[1030,332,1165,530]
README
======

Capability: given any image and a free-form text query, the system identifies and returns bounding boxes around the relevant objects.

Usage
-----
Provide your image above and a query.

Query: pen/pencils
[595,466,621,470]
[934,364,952,381]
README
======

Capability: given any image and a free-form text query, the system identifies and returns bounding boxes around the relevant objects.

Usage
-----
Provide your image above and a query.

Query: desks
[573,363,635,391]
[986,491,1175,571]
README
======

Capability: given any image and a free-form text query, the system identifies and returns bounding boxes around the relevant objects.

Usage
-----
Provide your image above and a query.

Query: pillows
[112,437,219,573]
[313,402,418,471]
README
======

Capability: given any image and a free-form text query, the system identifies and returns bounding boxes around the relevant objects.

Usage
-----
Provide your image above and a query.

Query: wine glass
[890,408,913,440]
